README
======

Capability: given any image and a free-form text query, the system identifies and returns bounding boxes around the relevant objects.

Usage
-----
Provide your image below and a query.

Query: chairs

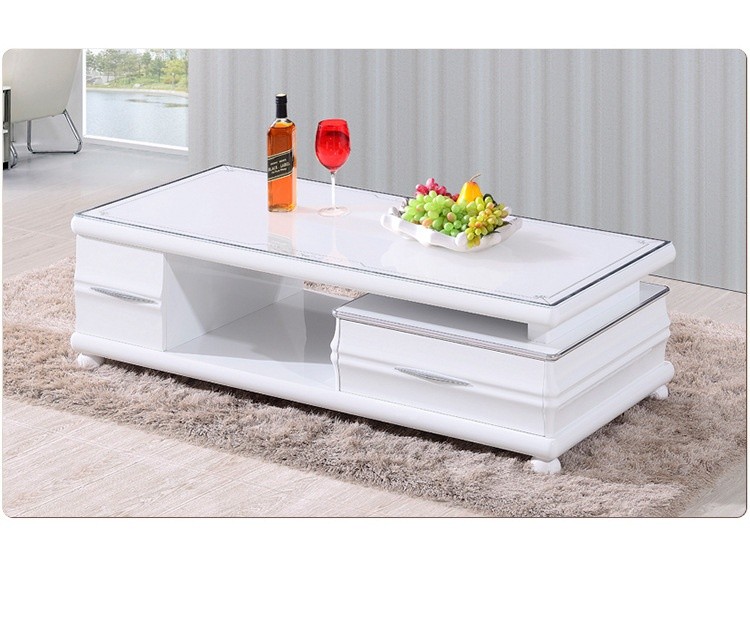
[2,50,82,155]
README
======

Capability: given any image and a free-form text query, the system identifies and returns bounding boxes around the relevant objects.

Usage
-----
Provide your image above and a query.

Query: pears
[457,174,484,207]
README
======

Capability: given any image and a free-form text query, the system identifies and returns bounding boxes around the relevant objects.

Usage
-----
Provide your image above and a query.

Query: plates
[380,207,522,252]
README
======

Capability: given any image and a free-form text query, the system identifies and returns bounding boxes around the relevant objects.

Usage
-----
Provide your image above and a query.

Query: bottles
[267,93,296,212]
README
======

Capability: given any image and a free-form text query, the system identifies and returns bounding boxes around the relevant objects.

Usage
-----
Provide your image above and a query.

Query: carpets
[1,253,746,518]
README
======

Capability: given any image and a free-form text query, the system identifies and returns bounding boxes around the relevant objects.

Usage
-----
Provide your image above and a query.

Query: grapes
[401,178,508,248]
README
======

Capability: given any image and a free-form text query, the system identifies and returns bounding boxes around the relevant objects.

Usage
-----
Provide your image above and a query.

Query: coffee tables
[72,162,677,474]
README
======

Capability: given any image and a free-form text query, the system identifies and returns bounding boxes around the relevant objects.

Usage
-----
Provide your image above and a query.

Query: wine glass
[315,118,350,217]
